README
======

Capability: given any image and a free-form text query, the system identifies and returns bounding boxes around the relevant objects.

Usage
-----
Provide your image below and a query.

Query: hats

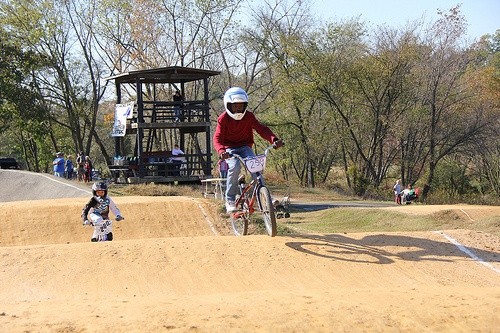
[56,152,61,154]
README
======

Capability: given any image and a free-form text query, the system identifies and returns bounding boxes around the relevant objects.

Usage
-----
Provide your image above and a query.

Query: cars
[0,157,23,169]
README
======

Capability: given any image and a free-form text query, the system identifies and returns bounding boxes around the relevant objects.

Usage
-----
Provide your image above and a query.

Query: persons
[172,144,184,177]
[52,152,92,183]
[81,182,122,242]
[393,180,414,205]
[218,157,228,191]
[214,87,283,213]
[173,91,183,123]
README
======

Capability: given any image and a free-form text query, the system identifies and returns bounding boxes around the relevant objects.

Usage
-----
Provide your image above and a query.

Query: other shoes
[225,201,238,214]
[271,197,279,212]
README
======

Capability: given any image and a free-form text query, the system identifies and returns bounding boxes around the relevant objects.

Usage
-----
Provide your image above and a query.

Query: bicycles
[82,217,124,241]
[218,142,285,237]
[76,162,88,183]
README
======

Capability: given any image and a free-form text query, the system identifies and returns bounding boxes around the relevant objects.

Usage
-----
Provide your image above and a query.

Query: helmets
[92,182,107,202]
[224,87,248,120]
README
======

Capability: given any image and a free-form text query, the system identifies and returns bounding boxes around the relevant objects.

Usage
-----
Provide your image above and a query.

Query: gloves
[274,140,283,147]
[85,220,88,224]
[116,215,122,221]
[221,152,230,159]
